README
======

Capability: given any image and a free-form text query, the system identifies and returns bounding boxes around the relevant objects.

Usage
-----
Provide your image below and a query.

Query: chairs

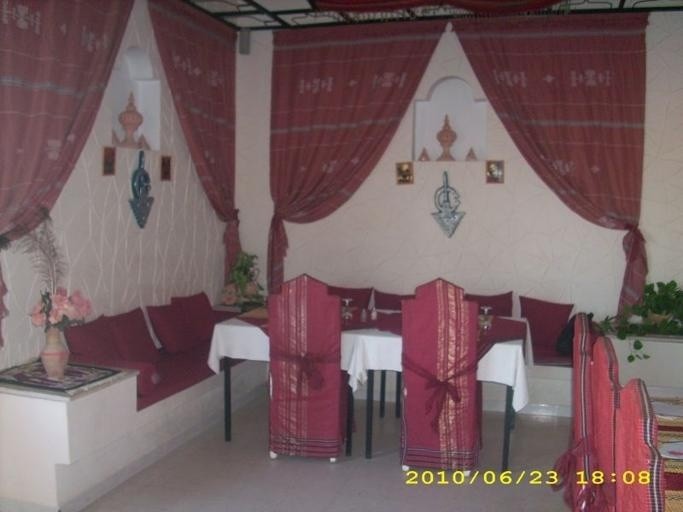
[401,278,481,471]
[266,274,350,463]
[550,314,683,512]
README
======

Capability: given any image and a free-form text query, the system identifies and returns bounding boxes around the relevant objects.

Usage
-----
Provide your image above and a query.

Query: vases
[40,326,70,380]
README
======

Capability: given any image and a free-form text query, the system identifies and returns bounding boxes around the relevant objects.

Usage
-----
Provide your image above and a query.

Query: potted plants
[601,281,683,362]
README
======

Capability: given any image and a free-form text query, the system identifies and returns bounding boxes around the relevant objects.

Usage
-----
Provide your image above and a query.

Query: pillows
[331,287,573,352]
[68,294,213,391]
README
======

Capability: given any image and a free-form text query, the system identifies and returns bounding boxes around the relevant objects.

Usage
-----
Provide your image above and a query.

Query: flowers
[12,206,91,330]
[220,251,264,305]
